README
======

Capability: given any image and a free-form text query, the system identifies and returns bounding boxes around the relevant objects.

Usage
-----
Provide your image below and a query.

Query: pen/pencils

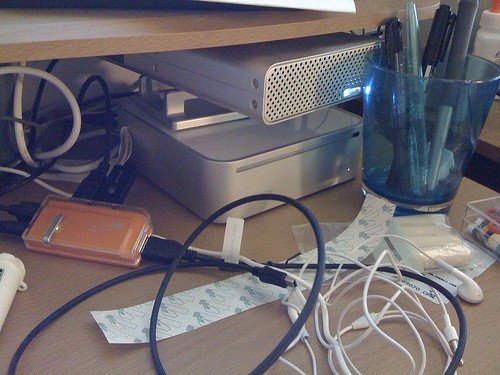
[406,1,427,196]
[386,17,411,197]
[419,3,457,106]
[424,1,480,196]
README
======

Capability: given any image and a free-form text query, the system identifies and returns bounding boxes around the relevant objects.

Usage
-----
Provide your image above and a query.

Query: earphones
[286,289,313,341]
[433,258,483,304]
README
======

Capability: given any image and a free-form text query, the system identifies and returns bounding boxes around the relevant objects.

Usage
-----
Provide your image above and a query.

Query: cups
[359,48,500,216]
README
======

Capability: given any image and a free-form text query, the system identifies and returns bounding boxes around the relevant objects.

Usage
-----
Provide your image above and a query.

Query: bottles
[473,0,500,66]
